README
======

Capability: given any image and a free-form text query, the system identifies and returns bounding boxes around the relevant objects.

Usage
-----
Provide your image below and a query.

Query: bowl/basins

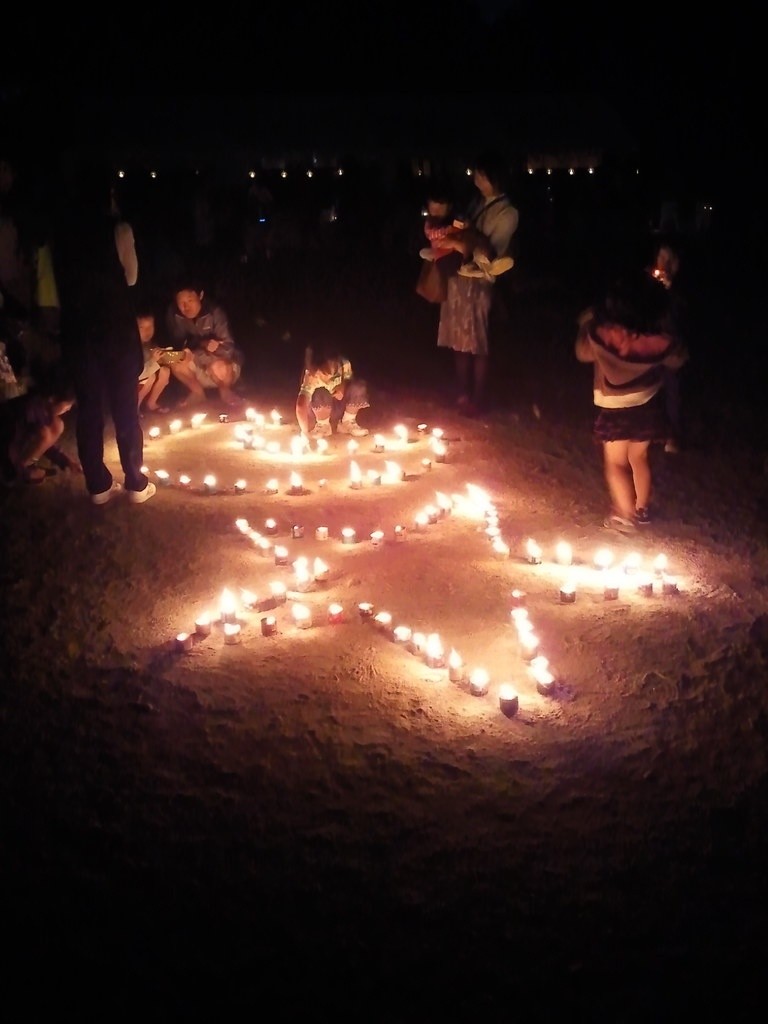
[151,348,185,365]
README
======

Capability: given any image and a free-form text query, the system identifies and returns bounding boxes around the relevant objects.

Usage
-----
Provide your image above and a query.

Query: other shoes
[665,438,680,454]
[222,394,245,406]
[22,465,58,485]
[137,406,170,419]
[487,257,513,276]
[457,263,485,278]
[175,398,206,408]
[455,393,482,418]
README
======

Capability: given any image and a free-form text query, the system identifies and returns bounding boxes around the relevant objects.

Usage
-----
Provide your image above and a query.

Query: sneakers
[92,481,122,504]
[305,422,332,438]
[603,514,633,533]
[633,507,651,525]
[337,420,369,437]
[128,482,157,503]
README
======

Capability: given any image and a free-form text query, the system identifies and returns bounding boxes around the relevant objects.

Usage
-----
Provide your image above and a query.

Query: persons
[0,160,36,314]
[36,159,156,505]
[291,343,371,440]
[189,175,342,239]
[162,280,244,409]
[135,311,170,419]
[0,379,74,482]
[639,234,693,454]
[577,282,685,529]
[417,141,520,415]
[422,189,513,278]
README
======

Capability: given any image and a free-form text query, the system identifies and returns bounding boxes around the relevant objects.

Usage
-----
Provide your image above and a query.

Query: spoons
[150,347,173,351]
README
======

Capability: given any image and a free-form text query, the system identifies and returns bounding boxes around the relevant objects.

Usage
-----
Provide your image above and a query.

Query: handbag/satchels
[416,256,447,304]
